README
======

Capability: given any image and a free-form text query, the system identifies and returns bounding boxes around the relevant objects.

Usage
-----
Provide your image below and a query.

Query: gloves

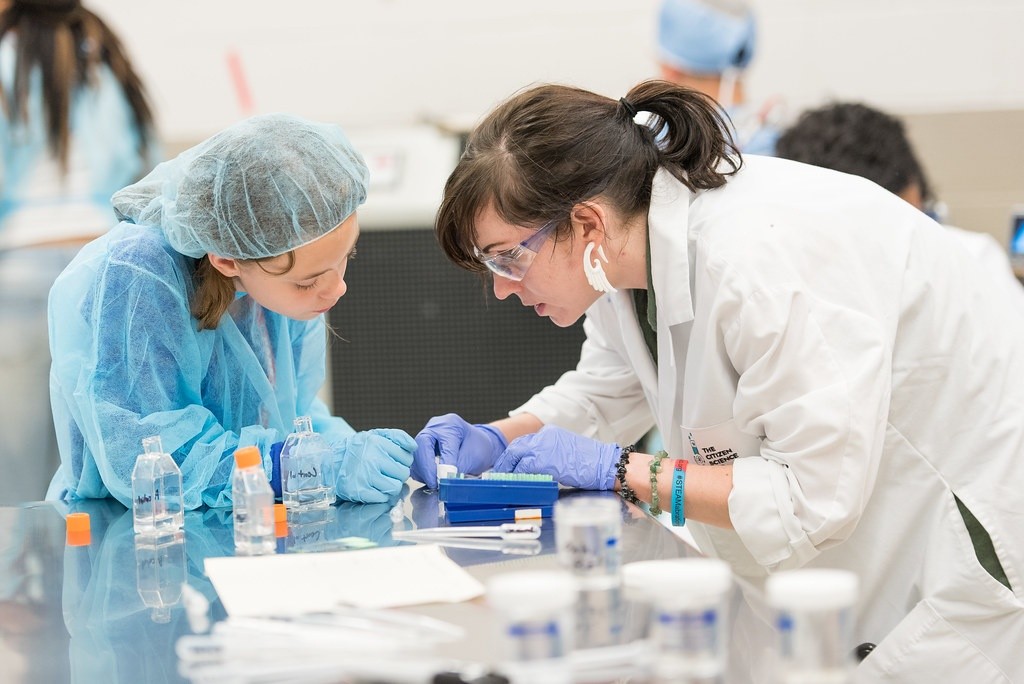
[287,429,418,504]
[493,424,622,491]
[410,413,509,490]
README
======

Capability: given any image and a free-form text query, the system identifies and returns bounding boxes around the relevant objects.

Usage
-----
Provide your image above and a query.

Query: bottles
[494,496,861,684]
[281,415,334,528]
[132,435,186,546]
[230,445,277,556]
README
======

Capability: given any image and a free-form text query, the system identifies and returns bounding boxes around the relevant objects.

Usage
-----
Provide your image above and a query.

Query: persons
[406,76,1024,684]
[45,115,420,505]
[0,1,931,247]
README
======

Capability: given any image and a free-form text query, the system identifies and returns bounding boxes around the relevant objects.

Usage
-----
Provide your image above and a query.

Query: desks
[0,491,864,684]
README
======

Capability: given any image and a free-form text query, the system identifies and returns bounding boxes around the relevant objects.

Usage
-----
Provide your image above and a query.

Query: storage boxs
[438,478,559,521]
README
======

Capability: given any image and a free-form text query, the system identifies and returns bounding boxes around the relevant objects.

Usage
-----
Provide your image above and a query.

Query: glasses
[473,184,601,281]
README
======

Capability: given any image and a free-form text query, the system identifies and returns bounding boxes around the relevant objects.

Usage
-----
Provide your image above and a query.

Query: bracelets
[616,440,686,526]
